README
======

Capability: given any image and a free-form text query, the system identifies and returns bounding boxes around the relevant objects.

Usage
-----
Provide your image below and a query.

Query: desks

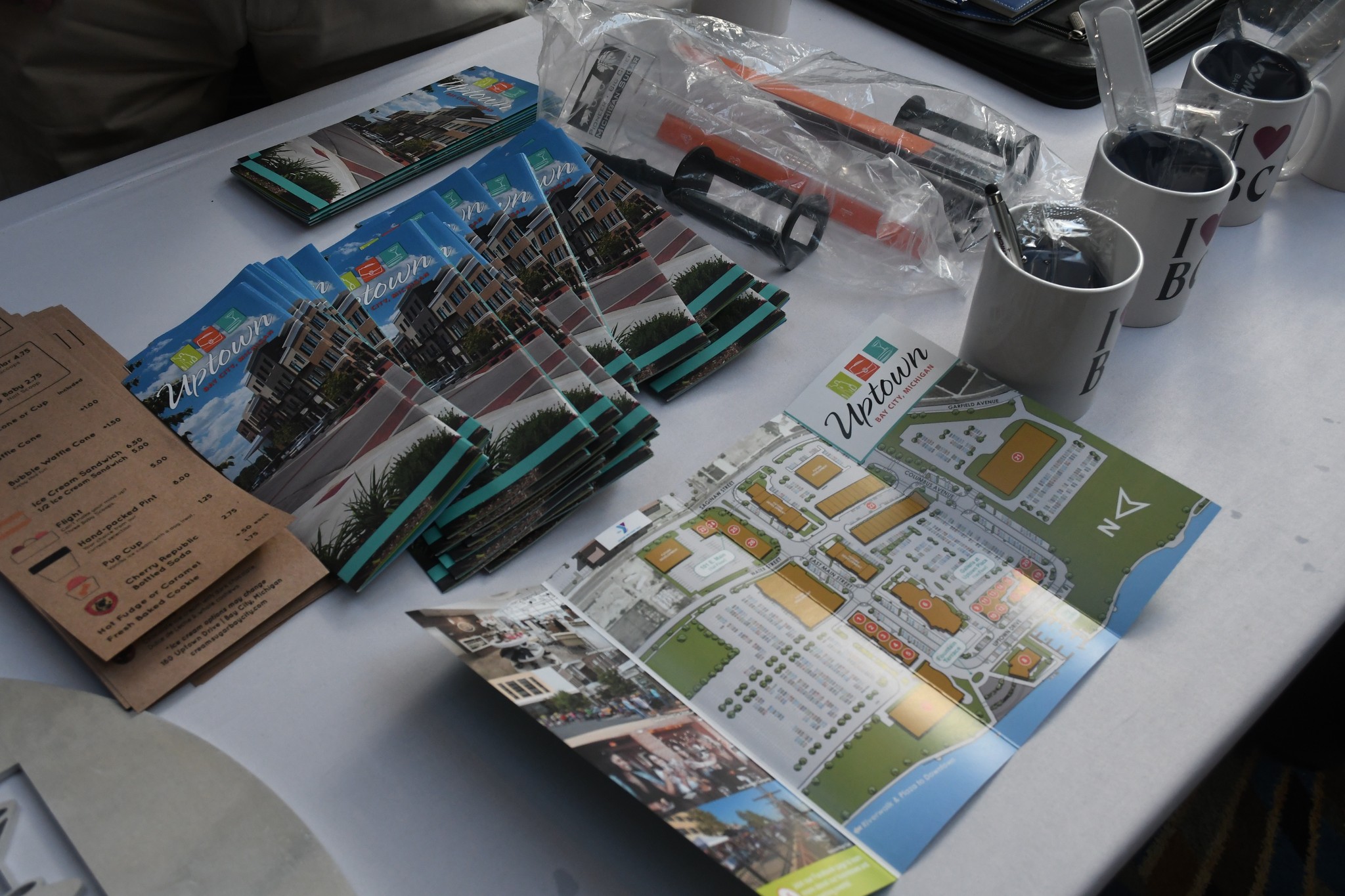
[0,0,1345,896]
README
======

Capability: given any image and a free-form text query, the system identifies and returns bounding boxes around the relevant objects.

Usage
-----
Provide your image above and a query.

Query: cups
[688,0,792,40]
[1171,45,1331,229]
[957,199,1145,424]
[1080,124,1239,330]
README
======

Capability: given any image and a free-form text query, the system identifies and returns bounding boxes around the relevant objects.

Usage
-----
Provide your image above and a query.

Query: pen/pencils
[984,183,1024,271]
